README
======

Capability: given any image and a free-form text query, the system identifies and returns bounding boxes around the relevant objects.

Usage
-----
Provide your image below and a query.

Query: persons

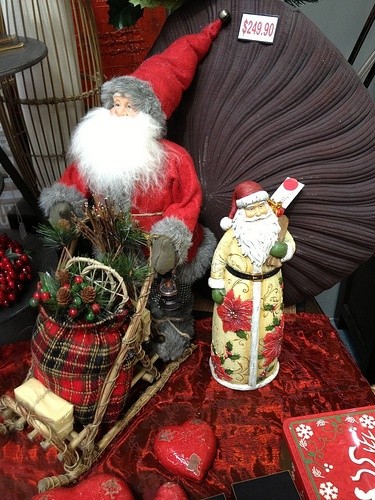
[37,19,222,361]
[207,181,295,391]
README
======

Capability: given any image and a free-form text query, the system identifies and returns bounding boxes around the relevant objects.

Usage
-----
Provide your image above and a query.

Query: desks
[0,297,375,500]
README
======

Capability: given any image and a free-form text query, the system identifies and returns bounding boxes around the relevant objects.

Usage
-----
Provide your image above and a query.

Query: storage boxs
[279,405,375,500]
